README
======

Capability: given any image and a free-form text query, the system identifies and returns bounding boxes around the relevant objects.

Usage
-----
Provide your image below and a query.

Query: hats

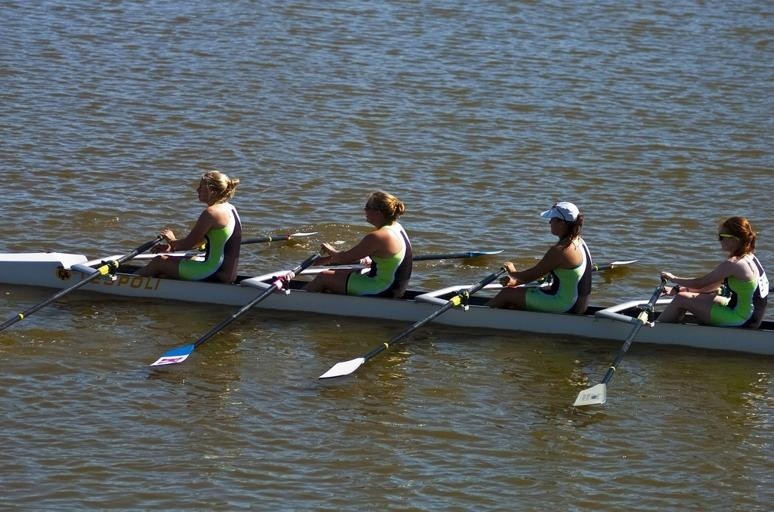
[540,202,581,221]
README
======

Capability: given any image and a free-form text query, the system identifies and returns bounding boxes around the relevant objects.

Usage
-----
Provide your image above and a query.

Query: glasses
[718,232,741,241]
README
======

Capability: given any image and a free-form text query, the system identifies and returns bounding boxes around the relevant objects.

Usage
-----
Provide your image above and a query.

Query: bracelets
[168,242,175,253]
[686,286,689,292]
[674,284,680,293]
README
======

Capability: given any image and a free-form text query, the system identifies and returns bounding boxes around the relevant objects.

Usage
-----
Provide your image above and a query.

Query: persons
[484,201,592,313]
[299,191,412,298]
[655,216,769,329]
[131,170,243,285]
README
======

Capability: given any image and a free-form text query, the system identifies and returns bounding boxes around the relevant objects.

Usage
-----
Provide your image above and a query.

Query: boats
[0,253,774,357]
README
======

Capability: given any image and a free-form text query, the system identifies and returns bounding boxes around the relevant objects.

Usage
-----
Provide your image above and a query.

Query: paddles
[149,245,327,365]
[318,268,508,380]
[156,232,318,251]
[501,260,638,283]
[573,276,668,405]
[322,249,503,266]
